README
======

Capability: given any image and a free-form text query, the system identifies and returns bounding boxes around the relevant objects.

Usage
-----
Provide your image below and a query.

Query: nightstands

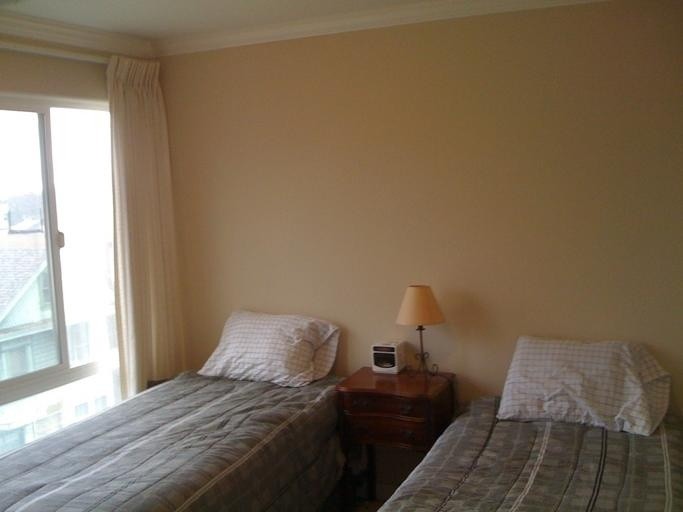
[336,365,456,502]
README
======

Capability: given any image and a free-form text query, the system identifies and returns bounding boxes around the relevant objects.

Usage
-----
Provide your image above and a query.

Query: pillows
[198,308,340,387]
[494,332,645,434]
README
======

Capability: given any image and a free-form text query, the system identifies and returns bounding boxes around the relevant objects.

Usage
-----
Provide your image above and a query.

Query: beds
[378,395,682,511]
[0,364,345,511]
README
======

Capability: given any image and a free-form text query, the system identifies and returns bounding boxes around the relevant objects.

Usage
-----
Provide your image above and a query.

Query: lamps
[395,286,447,385]
